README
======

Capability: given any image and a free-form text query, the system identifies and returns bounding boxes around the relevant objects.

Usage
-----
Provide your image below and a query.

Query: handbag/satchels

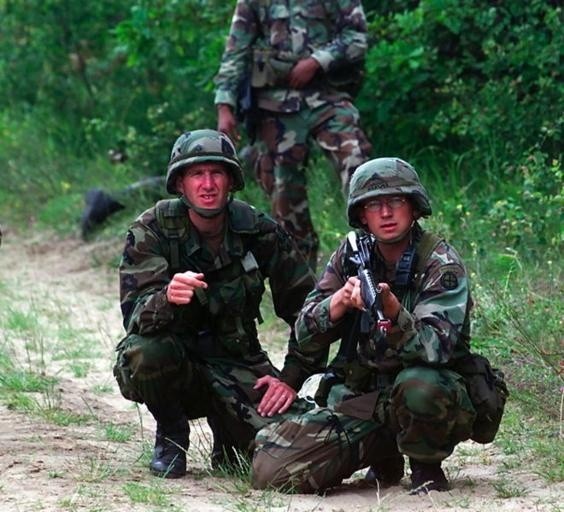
[466,350,509,443]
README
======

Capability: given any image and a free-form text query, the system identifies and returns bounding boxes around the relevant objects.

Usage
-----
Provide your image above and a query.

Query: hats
[347,158,430,229]
[165,129,244,195]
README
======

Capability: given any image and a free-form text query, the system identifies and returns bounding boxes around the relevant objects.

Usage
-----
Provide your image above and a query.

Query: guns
[343,231,392,336]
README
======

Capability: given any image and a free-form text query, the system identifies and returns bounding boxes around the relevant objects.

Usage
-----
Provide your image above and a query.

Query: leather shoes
[149,424,188,477]
[210,436,253,470]
[365,457,402,491]
[412,460,450,491]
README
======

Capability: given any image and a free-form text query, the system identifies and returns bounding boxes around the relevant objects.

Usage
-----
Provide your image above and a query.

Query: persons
[212,0,373,276]
[250,155,480,493]
[112,129,331,481]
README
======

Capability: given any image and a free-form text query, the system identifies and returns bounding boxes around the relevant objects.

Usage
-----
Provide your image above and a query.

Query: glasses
[361,195,408,213]
